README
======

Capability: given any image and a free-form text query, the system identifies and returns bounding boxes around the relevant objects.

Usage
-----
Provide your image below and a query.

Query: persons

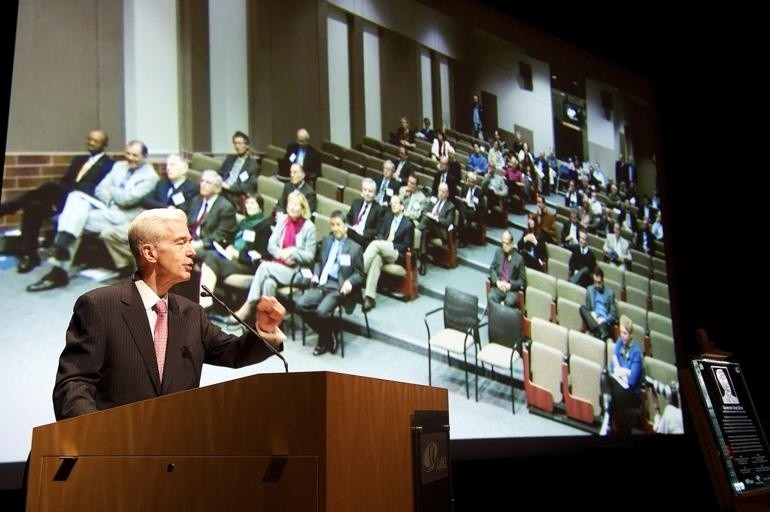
[647,381,684,434]
[598,314,645,436]
[50,207,288,422]
[1,92,664,356]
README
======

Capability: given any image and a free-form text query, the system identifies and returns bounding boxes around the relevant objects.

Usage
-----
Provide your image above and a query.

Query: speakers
[601,89,613,107]
[516,63,533,91]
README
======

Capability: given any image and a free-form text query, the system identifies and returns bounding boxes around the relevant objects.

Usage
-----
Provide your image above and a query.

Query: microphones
[197,283,290,372]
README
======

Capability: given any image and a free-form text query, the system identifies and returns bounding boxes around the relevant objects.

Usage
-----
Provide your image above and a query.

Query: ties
[377,178,388,204]
[319,239,340,286]
[471,188,472,201]
[154,299,168,381]
[404,192,411,212]
[120,171,131,188]
[435,200,443,215]
[356,204,368,226]
[441,174,445,183]
[192,203,208,240]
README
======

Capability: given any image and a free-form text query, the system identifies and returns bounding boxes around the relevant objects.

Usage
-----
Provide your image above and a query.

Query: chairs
[187,129,510,358]
[422,193,679,432]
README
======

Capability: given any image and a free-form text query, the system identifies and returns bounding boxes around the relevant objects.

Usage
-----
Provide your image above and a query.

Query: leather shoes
[441,230,448,243]
[224,313,241,326]
[314,340,327,356]
[459,239,467,248]
[17,232,69,292]
[419,259,428,276]
[0,197,9,218]
[599,326,611,340]
[362,295,375,312]
